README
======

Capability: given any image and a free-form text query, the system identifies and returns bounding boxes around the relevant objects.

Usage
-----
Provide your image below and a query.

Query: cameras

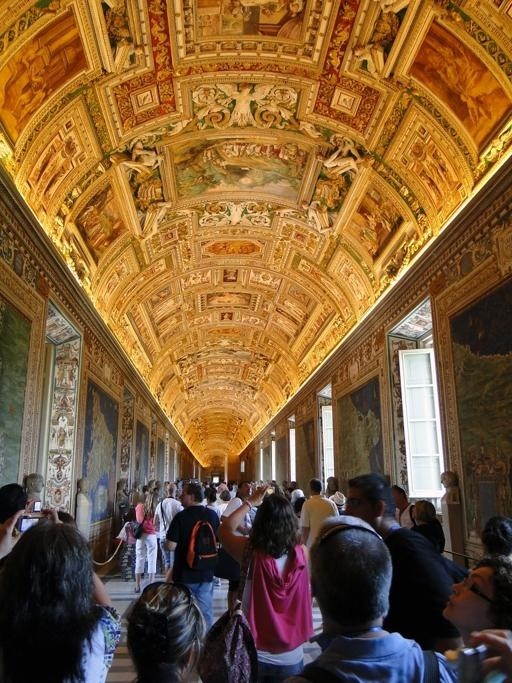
[458,645,487,683]
[257,487,275,498]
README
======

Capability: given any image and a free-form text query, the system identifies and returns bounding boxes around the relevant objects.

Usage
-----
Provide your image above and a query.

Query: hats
[329,491,347,509]
[291,489,305,504]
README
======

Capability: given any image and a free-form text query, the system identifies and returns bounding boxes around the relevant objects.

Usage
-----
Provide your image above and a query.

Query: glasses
[460,576,493,605]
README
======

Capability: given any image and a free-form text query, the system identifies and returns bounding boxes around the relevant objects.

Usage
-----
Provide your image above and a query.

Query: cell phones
[17,513,52,533]
[32,501,43,513]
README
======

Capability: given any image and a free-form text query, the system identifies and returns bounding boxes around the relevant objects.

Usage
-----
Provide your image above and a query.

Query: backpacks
[188,512,218,570]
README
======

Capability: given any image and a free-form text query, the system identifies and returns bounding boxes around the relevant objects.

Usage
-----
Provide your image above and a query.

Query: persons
[0,476,512,683]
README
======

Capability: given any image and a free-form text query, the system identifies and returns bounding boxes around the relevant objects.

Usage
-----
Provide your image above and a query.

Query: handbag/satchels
[132,522,143,538]
[215,546,234,578]
[197,609,258,683]
